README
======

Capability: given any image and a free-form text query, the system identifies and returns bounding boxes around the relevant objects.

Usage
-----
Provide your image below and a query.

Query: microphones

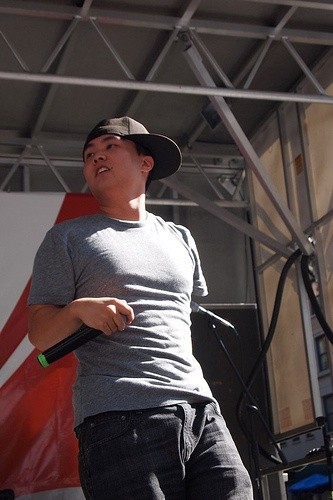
[36,313,127,368]
[190,301,238,336]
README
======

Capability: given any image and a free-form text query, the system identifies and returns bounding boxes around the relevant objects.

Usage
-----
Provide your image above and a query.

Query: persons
[24,116,254,500]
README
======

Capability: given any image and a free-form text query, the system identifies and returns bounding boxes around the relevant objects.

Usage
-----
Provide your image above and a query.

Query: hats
[82,116,182,183]
